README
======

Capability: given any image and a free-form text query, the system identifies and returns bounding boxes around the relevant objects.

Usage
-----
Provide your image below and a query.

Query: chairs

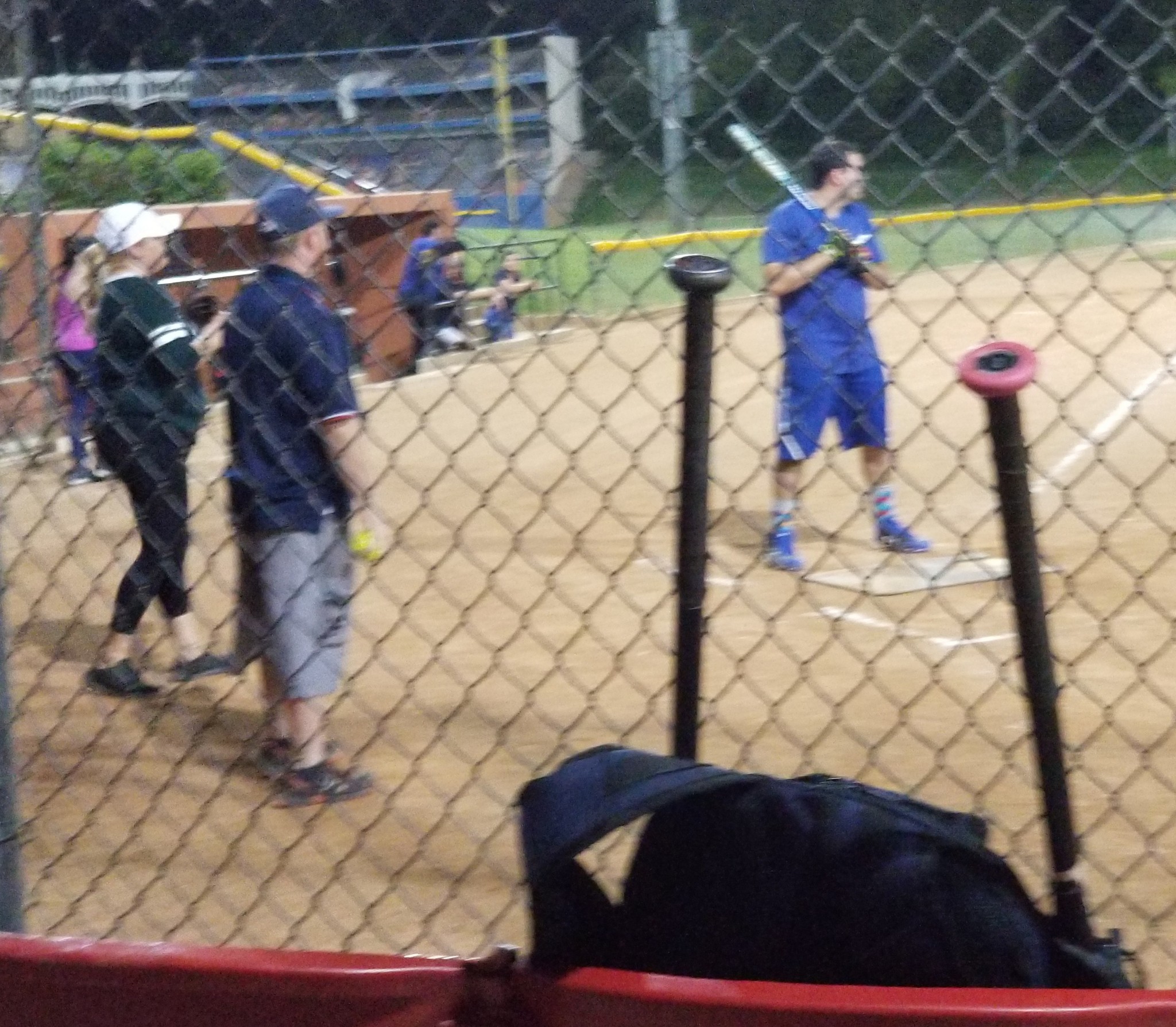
[0,934,1176,1027]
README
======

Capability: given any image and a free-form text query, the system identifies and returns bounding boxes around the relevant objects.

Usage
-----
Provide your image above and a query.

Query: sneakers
[879,525,927,553]
[768,529,801,571]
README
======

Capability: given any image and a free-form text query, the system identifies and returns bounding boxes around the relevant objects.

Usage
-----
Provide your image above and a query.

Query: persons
[220,184,369,810]
[93,201,238,694]
[51,235,114,485]
[483,251,534,341]
[397,222,471,359]
[760,140,929,570]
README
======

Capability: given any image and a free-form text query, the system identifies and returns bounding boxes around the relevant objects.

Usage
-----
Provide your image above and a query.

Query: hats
[94,203,185,252]
[255,186,340,243]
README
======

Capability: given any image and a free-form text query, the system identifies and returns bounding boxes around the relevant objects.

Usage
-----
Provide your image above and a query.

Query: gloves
[818,228,875,276]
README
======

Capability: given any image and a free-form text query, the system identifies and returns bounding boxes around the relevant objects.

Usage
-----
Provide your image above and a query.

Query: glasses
[840,160,868,177]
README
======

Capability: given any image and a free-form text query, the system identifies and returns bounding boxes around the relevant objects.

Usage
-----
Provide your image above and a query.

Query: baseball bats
[658,252,734,762]
[728,123,869,275]
[956,342,1090,936]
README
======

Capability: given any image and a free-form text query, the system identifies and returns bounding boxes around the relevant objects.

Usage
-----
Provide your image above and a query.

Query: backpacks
[515,742,1147,993]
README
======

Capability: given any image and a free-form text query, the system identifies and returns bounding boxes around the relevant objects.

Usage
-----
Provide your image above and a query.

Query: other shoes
[175,655,231,684]
[87,661,159,697]
[259,734,344,781]
[94,461,113,479]
[278,763,375,807]
[68,466,93,484]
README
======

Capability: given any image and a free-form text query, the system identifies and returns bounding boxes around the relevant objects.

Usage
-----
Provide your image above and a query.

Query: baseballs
[354,532,383,562]
